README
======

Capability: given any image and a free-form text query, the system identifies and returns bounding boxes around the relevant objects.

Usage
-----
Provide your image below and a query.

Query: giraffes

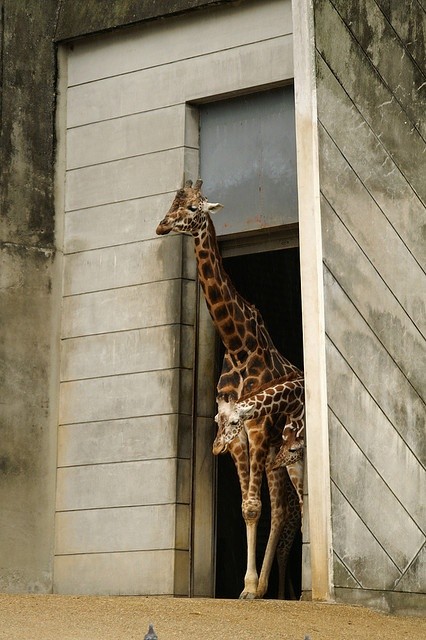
[270,427,306,534]
[212,370,304,456]
[155,179,306,600]
